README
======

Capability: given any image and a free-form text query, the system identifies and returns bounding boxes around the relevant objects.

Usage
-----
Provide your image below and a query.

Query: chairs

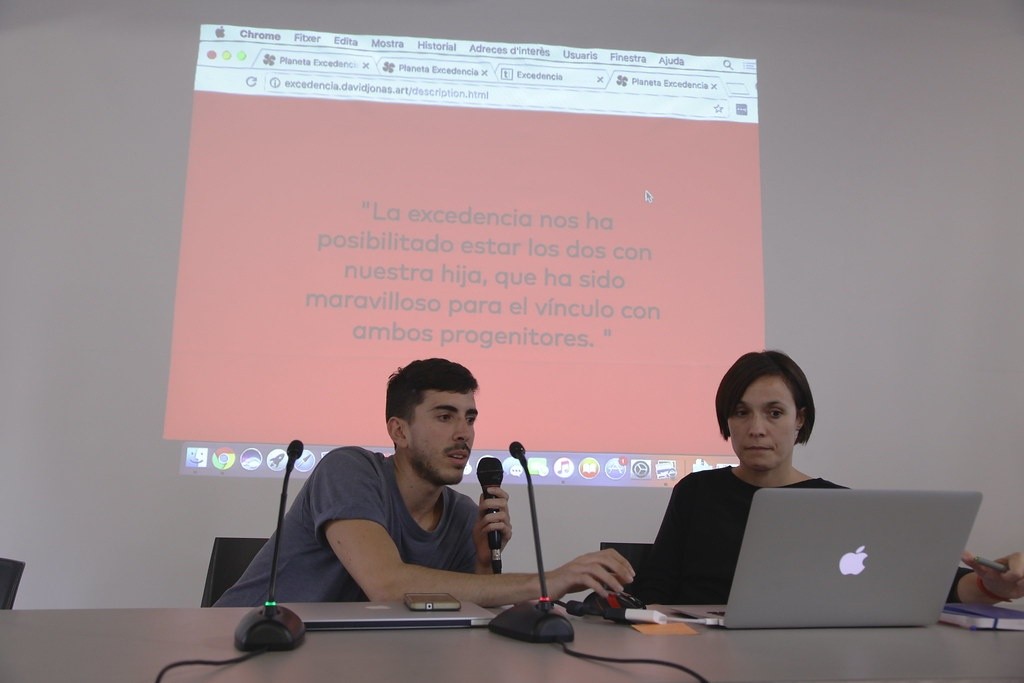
[0,557,26,610]
[600,543,652,570]
[200,537,270,607]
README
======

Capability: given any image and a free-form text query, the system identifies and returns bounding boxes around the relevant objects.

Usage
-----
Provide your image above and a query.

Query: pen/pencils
[974,555,1007,573]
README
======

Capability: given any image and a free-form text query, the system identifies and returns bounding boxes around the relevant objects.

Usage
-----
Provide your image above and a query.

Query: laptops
[277,599,497,631]
[672,488,984,628]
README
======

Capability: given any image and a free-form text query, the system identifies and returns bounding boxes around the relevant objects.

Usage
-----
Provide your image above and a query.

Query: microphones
[477,457,504,574]
[233,440,305,652]
[488,441,574,644]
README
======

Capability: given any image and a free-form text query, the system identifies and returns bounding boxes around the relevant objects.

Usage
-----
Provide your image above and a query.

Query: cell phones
[404,593,461,610]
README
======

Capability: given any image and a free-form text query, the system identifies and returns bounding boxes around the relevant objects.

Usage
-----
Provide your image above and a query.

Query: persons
[208,354,638,609]
[629,346,1023,609]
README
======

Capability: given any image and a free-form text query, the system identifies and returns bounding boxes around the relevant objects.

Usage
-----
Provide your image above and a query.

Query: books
[939,601,1024,633]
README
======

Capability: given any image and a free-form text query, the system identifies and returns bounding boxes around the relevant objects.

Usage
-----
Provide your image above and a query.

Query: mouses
[583,589,647,616]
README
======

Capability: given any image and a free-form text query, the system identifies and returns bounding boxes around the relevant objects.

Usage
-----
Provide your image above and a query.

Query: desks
[0,608,1024,683]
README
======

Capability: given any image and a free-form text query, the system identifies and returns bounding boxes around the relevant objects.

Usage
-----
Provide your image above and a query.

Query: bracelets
[975,572,1013,603]
